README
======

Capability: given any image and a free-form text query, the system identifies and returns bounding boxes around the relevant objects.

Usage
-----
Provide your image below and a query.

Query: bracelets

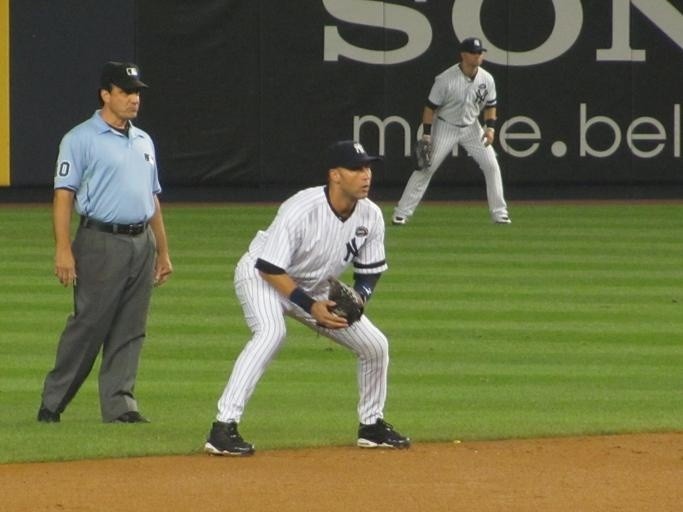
[289,286,314,314]
[486,119,495,127]
[424,122,433,135]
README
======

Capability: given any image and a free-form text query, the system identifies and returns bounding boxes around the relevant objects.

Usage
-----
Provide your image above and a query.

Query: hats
[330,140,380,169]
[463,38,487,53]
[100,62,149,91]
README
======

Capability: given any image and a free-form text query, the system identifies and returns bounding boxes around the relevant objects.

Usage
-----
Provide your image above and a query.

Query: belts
[81,215,143,235]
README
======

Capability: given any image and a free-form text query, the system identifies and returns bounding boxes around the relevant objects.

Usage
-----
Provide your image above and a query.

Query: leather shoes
[38,401,59,421]
[117,412,149,423]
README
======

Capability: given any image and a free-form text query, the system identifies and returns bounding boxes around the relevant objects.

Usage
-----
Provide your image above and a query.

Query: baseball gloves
[316,277,364,329]
[414,140,431,170]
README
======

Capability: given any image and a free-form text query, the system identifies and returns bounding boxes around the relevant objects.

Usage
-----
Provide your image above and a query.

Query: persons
[388,37,512,223]
[39,59,173,425]
[201,138,411,457]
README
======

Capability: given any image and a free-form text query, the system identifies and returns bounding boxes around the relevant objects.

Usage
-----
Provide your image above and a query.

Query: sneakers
[496,215,511,223]
[205,421,255,455]
[392,215,405,224]
[356,418,409,448]
